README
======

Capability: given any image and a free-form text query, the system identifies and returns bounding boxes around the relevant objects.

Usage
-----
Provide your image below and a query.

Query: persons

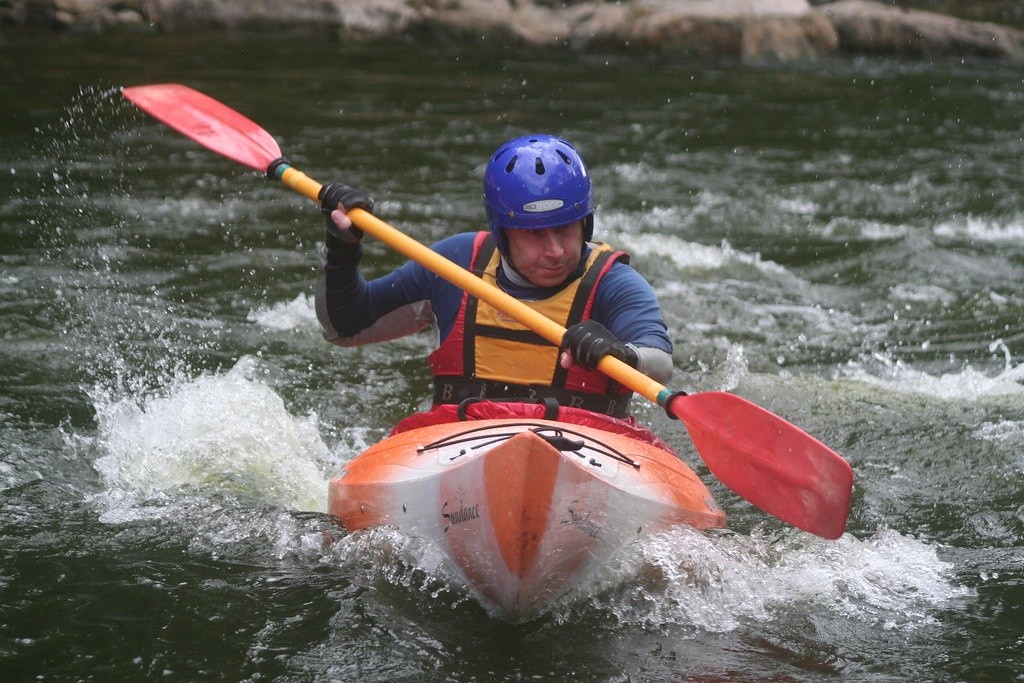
[314,134,675,425]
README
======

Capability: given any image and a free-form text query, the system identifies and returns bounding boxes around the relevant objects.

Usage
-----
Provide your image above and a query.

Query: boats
[325,397,727,622]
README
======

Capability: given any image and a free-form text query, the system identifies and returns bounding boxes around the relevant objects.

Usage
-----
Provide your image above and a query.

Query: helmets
[483,134,593,255]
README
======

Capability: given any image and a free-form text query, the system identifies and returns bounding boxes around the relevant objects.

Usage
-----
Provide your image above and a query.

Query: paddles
[119,82,853,541]
